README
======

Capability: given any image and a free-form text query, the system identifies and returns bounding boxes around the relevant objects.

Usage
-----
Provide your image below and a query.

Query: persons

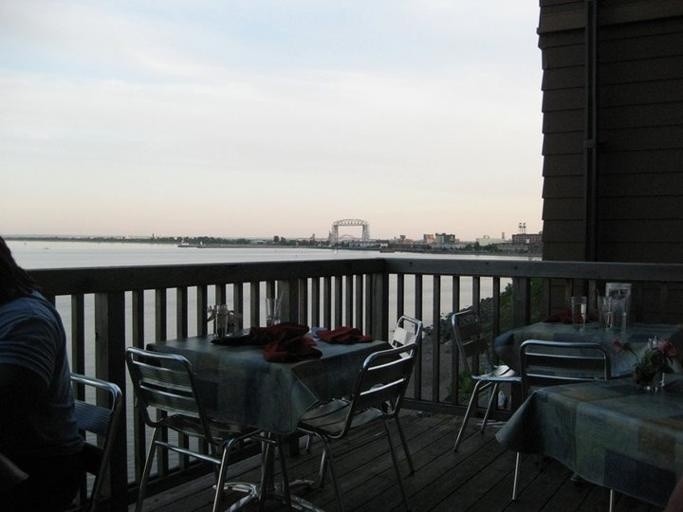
[0,235,84,512]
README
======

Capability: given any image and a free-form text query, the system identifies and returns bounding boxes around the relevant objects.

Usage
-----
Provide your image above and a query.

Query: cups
[264,297,282,326]
[570,294,627,335]
[212,304,229,340]
